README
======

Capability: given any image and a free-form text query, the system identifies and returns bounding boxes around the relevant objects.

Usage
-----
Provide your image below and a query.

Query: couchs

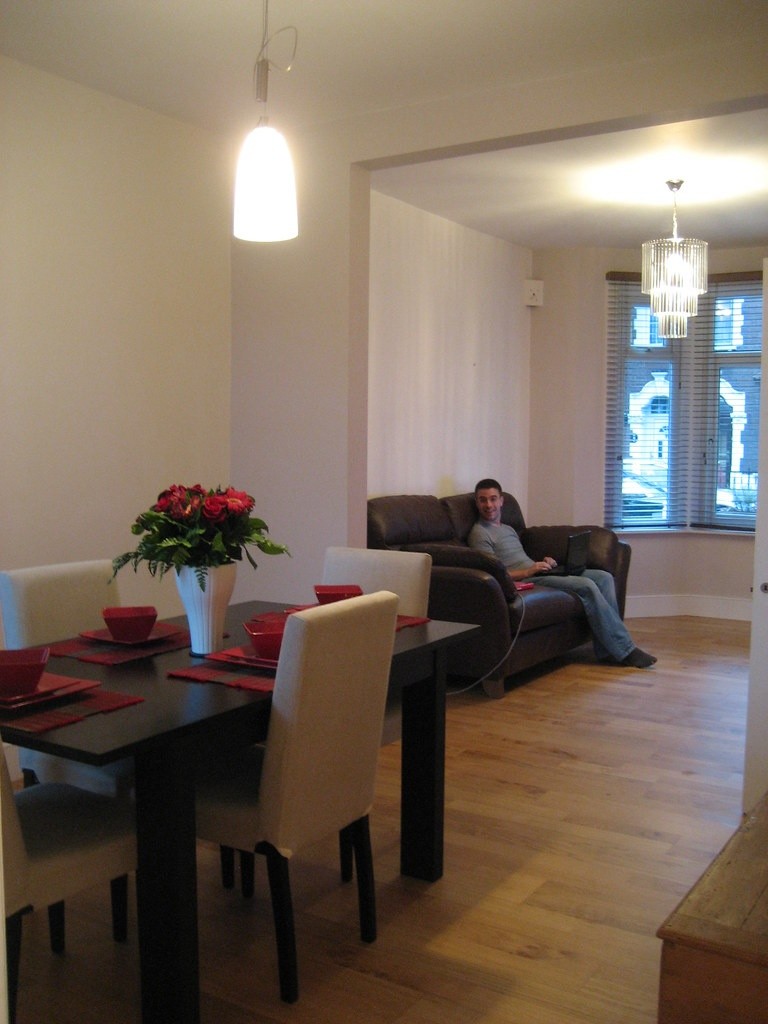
[368,491,632,698]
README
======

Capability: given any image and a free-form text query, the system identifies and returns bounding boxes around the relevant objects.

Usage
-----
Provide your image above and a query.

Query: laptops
[534,531,593,577]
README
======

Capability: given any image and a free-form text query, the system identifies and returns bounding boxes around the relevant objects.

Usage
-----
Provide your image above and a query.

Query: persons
[467,479,658,667]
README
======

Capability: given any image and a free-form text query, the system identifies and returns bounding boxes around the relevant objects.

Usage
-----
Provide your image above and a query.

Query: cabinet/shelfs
[655,796,768,1024]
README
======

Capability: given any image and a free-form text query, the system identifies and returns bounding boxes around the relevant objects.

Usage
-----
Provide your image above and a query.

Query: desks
[0,601,482,1024]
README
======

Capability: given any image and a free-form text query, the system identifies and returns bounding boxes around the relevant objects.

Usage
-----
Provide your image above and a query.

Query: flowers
[108,484,292,591]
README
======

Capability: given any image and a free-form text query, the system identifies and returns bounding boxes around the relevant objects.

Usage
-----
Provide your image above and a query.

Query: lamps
[641,179,711,338]
[233,0,298,242]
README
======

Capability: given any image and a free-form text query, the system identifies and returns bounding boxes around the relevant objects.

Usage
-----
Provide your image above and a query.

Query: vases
[176,560,236,654]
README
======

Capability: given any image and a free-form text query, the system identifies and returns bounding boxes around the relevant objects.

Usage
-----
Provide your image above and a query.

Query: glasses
[477,496,501,503]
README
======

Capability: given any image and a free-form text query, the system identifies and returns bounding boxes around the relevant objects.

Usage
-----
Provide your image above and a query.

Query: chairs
[196,590,397,1000]
[0,560,231,939]
[0,734,140,1024]
[239,546,432,897]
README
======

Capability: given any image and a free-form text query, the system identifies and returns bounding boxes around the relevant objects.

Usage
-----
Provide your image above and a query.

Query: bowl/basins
[103,606,158,641]
[243,620,285,660]
[0,647,51,694]
[313,584,363,604]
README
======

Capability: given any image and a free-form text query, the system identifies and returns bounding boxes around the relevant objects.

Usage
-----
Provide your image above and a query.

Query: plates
[205,645,278,669]
[79,621,184,645]
[0,673,101,710]
